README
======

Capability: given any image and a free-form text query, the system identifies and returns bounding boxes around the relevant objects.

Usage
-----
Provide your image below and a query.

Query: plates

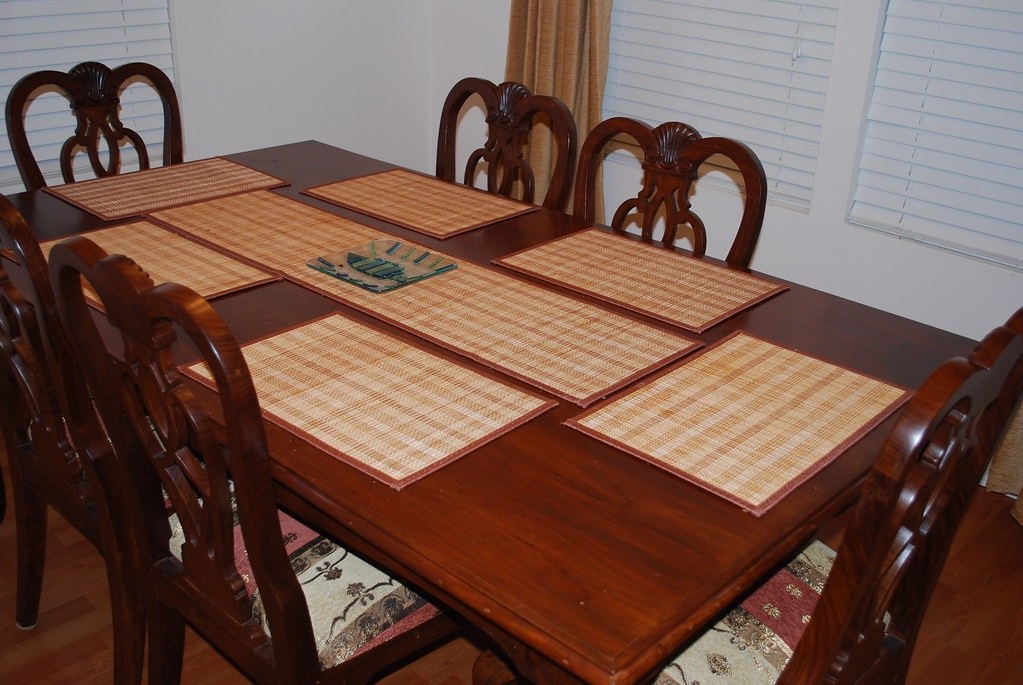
[306,240,457,293]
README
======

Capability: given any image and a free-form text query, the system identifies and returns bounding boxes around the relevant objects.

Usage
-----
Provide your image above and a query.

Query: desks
[0,140,977,685]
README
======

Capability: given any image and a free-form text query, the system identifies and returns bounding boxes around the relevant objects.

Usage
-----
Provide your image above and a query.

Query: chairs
[436,77,577,215]
[0,192,149,685]
[47,249,468,685]
[6,60,183,194]
[655,308,1023,685]
[574,118,766,265]
